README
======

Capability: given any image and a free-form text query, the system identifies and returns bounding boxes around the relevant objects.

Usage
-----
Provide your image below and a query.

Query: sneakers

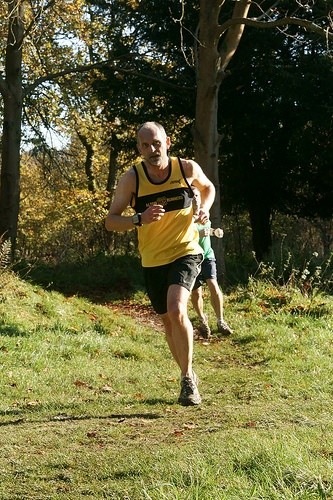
[217,321,233,335]
[178,371,201,406]
[200,314,211,339]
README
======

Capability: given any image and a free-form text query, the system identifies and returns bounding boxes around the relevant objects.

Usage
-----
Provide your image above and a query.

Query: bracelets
[212,229,215,236]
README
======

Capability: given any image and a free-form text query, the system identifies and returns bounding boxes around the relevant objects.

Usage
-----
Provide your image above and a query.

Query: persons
[105,122,215,406]
[189,186,232,338]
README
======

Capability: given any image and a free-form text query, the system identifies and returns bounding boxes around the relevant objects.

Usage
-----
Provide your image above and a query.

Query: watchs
[132,212,143,227]
